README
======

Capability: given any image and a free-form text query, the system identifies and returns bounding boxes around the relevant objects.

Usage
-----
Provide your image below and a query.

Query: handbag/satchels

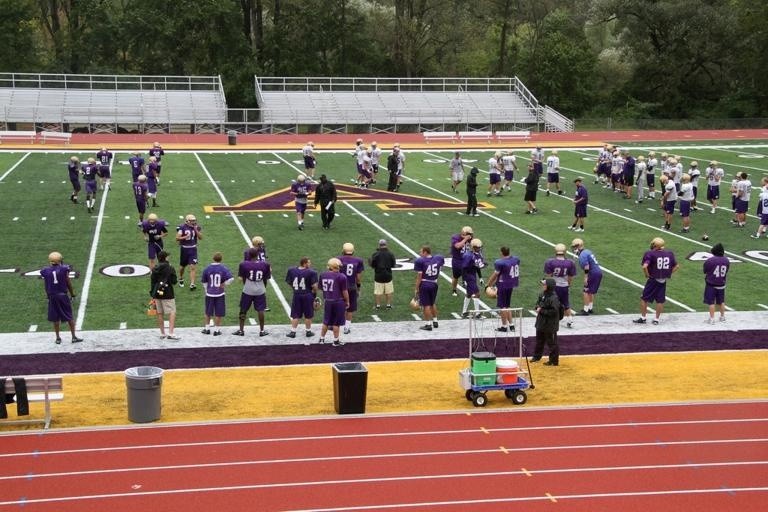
[559,301,565,320]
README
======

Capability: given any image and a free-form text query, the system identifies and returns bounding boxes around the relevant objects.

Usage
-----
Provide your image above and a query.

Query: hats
[736,172,743,177]
[540,279,546,285]
[157,250,171,258]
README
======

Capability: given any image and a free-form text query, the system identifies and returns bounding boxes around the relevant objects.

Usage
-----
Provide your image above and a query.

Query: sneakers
[495,326,515,332]
[467,214,479,217]
[568,224,584,232]
[661,221,689,233]
[286,326,350,346]
[690,205,768,239]
[452,281,487,320]
[704,317,725,324]
[160,333,181,340]
[525,209,537,215]
[260,331,269,336]
[487,187,511,196]
[355,179,376,190]
[568,309,594,328]
[388,180,403,192]
[201,329,244,336]
[298,222,304,230]
[558,191,565,194]
[373,304,392,311]
[592,180,631,199]
[56,337,83,344]
[547,191,550,196]
[71,183,111,213]
[178,278,196,289]
[634,196,655,204]
[633,318,658,325]
[530,356,558,366]
[419,322,438,331]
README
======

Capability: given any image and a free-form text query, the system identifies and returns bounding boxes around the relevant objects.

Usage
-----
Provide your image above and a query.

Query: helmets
[650,237,665,250]
[603,144,620,156]
[147,213,197,227]
[483,286,498,298]
[313,297,324,310]
[555,238,584,254]
[494,149,513,159]
[49,252,62,265]
[356,138,377,156]
[461,226,484,252]
[131,142,161,182]
[536,144,558,155]
[394,143,400,156]
[252,235,264,248]
[327,238,387,273]
[296,174,306,185]
[409,296,420,313]
[71,147,107,164]
[637,151,718,182]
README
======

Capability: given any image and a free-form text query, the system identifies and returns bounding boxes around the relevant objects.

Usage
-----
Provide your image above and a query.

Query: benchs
[38,131,72,144]
[0,376,65,430]
[2,88,227,124]
[259,88,536,126]
[423,131,457,144]
[0,130,36,144]
[459,131,493,145]
[495,131,532,143]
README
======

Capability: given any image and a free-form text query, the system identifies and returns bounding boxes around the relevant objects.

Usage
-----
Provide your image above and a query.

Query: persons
[303,141,317,179]
[201,253,234,336]
[175,215,204,289]
[314,175,337,230]
[233,236,271,336]
[368,239,396,311]
[568,178,589,232]
[633,237,678,324]
[387,143,405,191]
[129,142,164,224]
[290,175,313,231]
[68,147,113,213]
[703,243,729,324]
[140,214,168,272]
[150,251,181,340]
[286,242,365,347]
[593,144,767,239]
[352,138,381,190]
[449,145,563,217]
[41,252,84,344]
[415,226,602,365]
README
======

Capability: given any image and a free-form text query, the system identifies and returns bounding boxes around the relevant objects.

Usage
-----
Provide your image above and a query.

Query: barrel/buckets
[471,351,496,388]
[496,360,518,385]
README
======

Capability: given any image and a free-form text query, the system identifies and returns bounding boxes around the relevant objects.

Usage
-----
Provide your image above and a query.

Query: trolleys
[459,306,535,406]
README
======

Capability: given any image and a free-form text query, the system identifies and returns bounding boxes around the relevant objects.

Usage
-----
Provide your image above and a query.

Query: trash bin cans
[228,131,236,145]
[333,362,368,414]
[125,366,163,423]
